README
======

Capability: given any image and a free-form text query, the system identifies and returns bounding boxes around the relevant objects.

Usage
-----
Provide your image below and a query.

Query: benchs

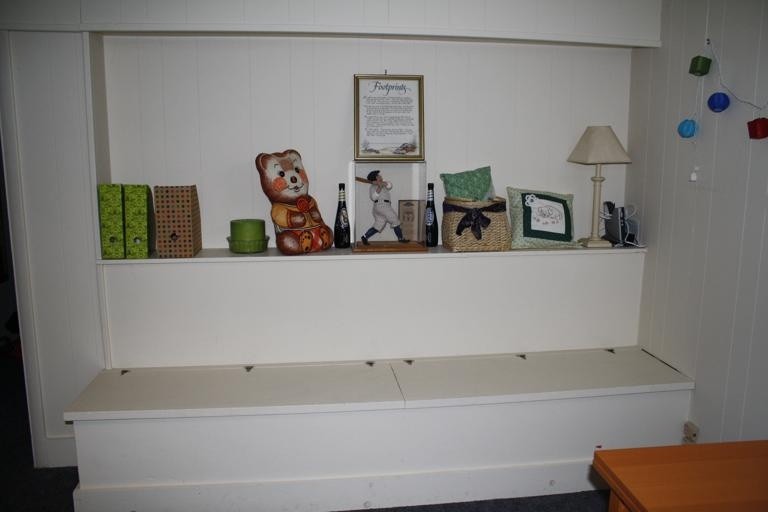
[61,345,697,511]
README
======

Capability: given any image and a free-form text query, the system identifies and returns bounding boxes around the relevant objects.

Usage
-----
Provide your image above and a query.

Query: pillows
[506,184,574,248]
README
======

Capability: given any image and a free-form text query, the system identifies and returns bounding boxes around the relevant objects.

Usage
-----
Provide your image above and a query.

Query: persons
[361,170,410,245]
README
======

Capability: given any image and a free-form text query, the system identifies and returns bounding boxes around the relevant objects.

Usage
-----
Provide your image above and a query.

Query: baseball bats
[355,177,387,185]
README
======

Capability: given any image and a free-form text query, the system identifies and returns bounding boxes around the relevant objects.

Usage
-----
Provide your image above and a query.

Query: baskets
[442,195,513,252]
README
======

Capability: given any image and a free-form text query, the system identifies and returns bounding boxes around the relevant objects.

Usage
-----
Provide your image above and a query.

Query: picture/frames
[354,72,425,163]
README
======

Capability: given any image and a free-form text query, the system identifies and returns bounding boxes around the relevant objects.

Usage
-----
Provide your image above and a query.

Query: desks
[592,440,768,512]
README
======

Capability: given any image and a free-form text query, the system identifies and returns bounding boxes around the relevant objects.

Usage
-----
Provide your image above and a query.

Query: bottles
[422,183,439,248]
[333,182,351,249]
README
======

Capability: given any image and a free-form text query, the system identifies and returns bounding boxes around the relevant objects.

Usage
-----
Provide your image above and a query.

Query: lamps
[567,126,631,249]
